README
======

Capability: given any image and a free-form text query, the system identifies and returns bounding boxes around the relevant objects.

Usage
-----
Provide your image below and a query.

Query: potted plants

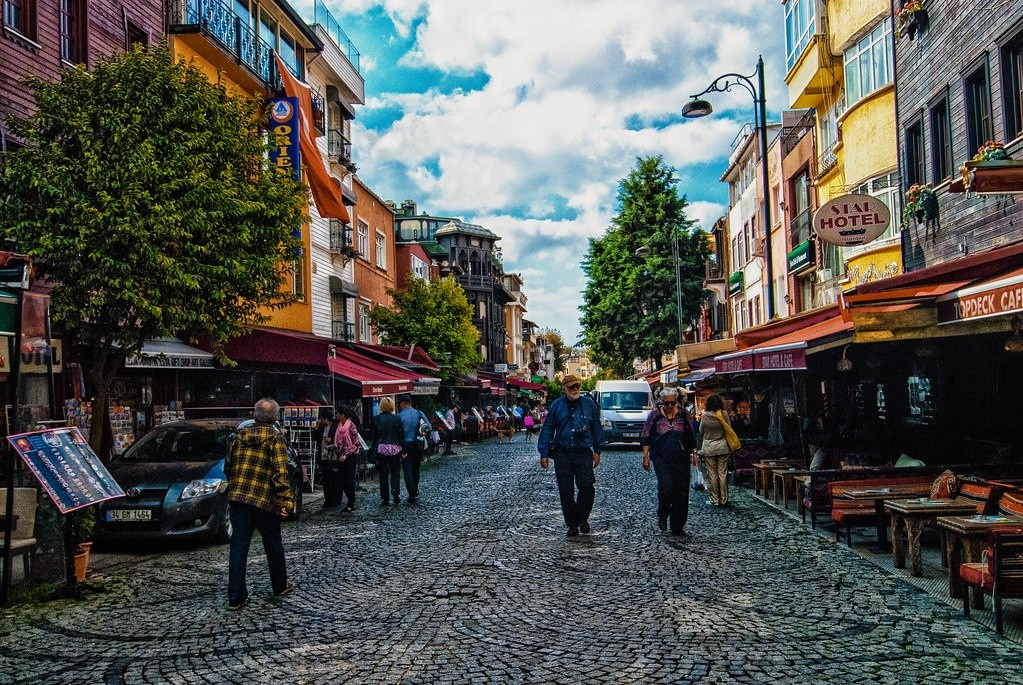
[35,491,96,582]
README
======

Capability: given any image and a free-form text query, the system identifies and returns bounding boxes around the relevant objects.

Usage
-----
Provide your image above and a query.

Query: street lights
[681,54,774,322]
[634,227,682,343]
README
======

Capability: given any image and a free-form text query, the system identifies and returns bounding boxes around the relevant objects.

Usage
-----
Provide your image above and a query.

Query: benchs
[732,443,1023,634]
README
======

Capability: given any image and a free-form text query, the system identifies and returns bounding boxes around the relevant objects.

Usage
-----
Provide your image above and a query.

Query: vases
[78,542,93,567]
[914,205,925,223]
[73,551,87,582]
[905,9,929,41]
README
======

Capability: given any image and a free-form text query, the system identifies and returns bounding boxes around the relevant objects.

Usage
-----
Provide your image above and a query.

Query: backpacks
[415,409,435,455]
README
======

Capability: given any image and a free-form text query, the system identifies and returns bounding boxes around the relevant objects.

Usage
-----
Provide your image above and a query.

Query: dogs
[525,425,542,440]
[497,427,515,444]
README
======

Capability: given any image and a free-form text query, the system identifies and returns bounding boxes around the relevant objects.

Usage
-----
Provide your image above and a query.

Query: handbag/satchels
[691,463,708,491]
[547,441,558,459]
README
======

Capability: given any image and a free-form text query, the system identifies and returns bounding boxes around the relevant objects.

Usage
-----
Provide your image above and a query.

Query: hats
[561,374,581,387]
[396,395,413,403]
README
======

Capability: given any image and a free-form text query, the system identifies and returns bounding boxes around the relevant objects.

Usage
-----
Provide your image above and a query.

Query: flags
[273,51,351,224]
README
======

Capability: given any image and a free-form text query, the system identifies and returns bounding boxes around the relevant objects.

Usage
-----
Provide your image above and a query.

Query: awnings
[101,337,215,369]
[198,323,547,397]
[646,265,1023,386]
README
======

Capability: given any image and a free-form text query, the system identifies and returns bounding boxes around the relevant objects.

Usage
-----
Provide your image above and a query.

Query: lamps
[836,345,853,372]
[779,202,788,211]
[784,295,793,305]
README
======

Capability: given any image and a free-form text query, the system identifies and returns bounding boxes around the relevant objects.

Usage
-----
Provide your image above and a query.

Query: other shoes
[227,599,248,611]
[566,526,580,536]
[407,490,420,503]
[706,501,719,506]
[673,529,686,536]
[580,521,590,533]
[381,488,401,505]
[276,581,296,595]
[342,506,355,512]
[323,500,340,511]
[659,518,667,530]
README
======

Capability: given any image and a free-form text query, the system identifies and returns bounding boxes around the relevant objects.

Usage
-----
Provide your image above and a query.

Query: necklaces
[662,406,675,427]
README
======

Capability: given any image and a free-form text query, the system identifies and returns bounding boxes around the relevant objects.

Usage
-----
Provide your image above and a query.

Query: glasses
[663,400,677,405]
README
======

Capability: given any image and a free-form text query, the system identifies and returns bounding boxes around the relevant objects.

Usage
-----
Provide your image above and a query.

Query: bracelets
[693,450,697,453]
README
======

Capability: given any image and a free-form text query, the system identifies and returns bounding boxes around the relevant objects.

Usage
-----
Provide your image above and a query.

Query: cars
[90,416,303,547]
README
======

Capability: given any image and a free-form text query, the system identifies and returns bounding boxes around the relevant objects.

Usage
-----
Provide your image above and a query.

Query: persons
[312,405,360,512]
[445,403,547,455]
[370,397,407,504]
[223,396,295,609]
[699,395,731,505]
[396,394,432,501]
[538,374,602,535]
[641,388,698,535]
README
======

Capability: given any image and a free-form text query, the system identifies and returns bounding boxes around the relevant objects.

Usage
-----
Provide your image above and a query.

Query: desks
[885,498,977,577]
[842,487,917,554]
[752,464,799,499]
[793,476,806,515]
[936,516,1023,609]
[771,470,805,509]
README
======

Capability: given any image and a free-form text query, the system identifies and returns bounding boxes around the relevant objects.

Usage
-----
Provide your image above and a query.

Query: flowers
[900,182,940,244]
[971,141,1012,162]
[888,0,921,43]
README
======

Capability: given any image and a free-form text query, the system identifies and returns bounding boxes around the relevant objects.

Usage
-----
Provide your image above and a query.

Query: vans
[592,380,656,450]
